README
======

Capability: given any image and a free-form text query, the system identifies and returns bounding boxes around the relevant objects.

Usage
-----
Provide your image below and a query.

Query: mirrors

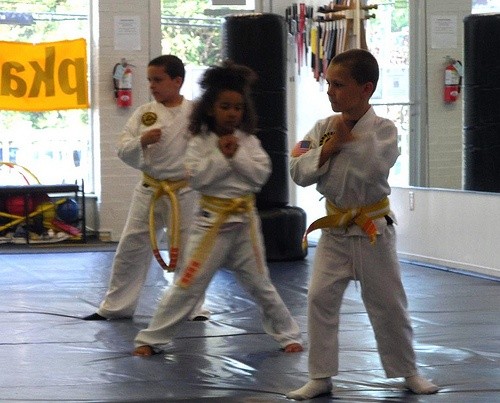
[363,0,500,197]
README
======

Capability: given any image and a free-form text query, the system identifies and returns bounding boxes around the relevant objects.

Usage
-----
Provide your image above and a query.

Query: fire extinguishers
[113,58,135,107]
[443,56,462,103]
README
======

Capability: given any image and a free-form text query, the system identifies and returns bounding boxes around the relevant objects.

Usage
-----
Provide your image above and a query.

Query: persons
[286,48,441,401]
[132,63,305,356]
[81,55,211,321]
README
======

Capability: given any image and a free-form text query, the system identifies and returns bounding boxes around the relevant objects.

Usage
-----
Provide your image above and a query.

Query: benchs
[1,178,87,246]
